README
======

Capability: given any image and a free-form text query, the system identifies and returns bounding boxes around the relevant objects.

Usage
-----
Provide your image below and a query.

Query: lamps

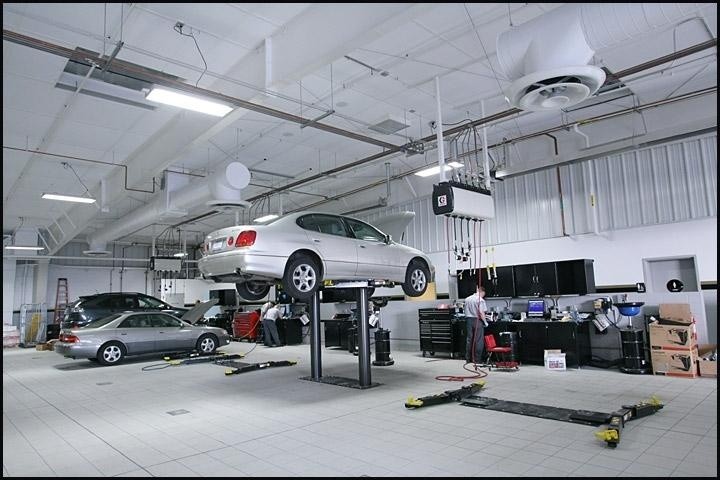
[144,81,235,119]
[40,189,96,207]
[413,147,465,179]
[5,243,46,252]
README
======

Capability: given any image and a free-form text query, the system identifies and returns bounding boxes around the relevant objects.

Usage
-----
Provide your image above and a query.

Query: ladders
[54,278,68,326]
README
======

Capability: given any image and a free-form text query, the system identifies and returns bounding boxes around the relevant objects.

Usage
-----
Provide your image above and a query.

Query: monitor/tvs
[527,300,547,317]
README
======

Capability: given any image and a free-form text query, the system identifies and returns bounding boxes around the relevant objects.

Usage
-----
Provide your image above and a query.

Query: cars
[199,209,435,304]
[54,298,232,367]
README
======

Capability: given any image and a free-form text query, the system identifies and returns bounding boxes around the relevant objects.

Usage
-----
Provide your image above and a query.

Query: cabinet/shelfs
[274,318,303,347]
[449,319,592,370]
[456,258,596,299]
[320,319,359,350]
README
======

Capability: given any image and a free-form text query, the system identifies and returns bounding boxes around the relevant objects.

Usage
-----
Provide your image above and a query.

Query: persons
[264,304,283,346]
[260,302,274,327]
[465,287,489,364]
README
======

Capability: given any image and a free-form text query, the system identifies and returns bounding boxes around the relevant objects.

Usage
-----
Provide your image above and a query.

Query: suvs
[59,290,206,329]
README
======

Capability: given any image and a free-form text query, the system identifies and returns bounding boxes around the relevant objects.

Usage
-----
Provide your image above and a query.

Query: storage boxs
[3,322,56,352]
[648,301,718,379]
